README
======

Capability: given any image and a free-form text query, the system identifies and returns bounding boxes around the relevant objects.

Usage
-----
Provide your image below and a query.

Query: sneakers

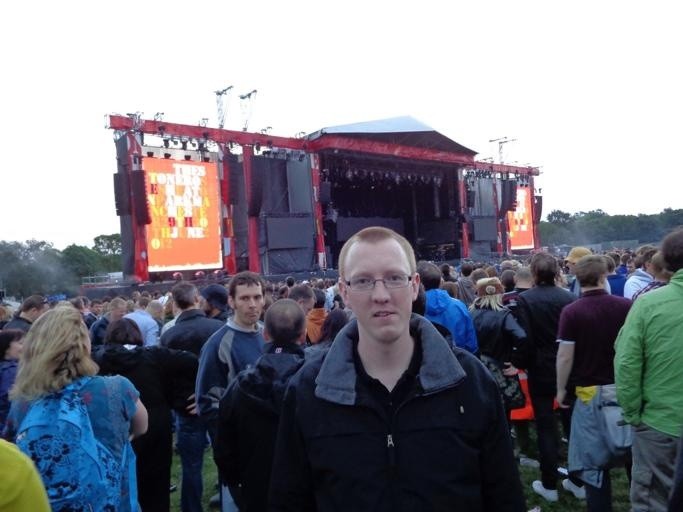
[519,454,541,469]
[561,476,588,501]
[561,436,569,443]
[531,479,559,503]
[513,448,518,460]
[510,429,517,439]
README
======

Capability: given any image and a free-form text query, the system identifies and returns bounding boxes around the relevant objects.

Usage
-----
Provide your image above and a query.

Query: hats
[199,283,230,313]
[563,246,594,265]
[476,276,506,297]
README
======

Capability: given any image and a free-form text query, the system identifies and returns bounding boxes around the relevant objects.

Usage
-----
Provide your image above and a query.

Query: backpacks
[14,374,129,512]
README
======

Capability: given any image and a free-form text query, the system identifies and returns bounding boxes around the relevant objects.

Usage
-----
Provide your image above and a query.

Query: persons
[265,226,529,510]
[2,245,683,512]
[613,230,683,512]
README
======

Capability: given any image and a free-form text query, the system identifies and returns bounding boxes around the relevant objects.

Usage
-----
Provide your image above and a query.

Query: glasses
[342,271,413,292]
